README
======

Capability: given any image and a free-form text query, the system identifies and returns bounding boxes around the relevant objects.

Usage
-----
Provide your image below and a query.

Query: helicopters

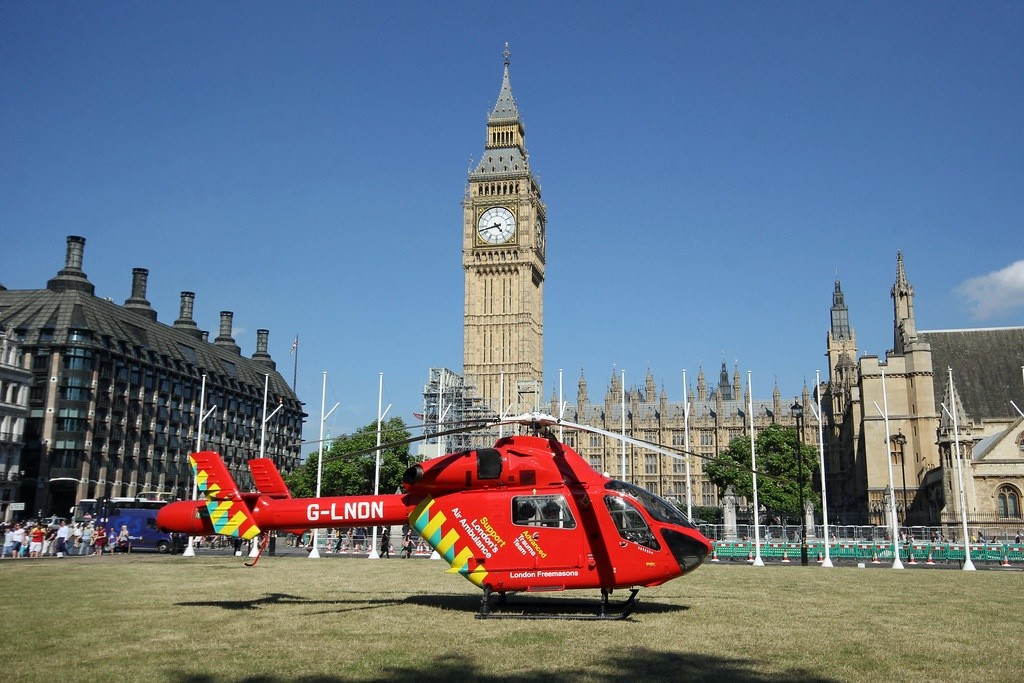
[154,411,798,622]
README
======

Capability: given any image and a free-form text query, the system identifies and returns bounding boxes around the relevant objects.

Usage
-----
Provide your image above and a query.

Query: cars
[41,517,68,531]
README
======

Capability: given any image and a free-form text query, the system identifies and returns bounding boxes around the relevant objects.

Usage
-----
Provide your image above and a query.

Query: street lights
[896,427,909,535]
[791,401,809,566]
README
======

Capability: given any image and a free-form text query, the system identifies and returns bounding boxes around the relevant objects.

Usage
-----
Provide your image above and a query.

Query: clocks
[477,206,515,244]
[535,215,543,251]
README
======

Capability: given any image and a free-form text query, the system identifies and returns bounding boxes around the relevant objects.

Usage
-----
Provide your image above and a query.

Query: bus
[69,497,188,554]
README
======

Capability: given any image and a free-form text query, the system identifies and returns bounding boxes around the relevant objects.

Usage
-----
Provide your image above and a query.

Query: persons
[722,529,1024,545]
[0,513,129,559]
[169,525,434,560]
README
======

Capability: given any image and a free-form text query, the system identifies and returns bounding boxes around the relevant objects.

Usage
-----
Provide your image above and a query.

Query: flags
[290,338,297,355]
